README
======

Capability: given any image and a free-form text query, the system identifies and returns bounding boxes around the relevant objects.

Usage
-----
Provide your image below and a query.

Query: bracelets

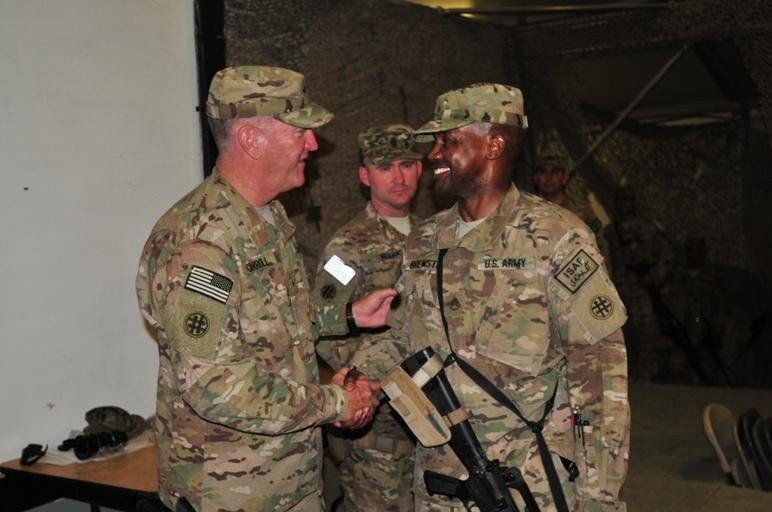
[344,301,358,335]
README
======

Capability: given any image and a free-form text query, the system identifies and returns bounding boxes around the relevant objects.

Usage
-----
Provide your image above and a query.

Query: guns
[399,347,517,511]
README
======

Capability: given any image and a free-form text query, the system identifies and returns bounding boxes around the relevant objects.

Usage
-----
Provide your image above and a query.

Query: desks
[0,425,159,512]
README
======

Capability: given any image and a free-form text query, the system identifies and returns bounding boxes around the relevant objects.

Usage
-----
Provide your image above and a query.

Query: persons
[136,64,383,511]
[309,119,435,511]
[329,79,631,511]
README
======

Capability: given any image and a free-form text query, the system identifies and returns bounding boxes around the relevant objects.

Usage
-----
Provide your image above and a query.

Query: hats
[206,65,335,129]
[83,405,147,441]
[411,82,528,143]
[356,123,424,165]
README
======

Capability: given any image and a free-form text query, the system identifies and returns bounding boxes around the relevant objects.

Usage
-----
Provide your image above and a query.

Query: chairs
[703,403,772,492]
